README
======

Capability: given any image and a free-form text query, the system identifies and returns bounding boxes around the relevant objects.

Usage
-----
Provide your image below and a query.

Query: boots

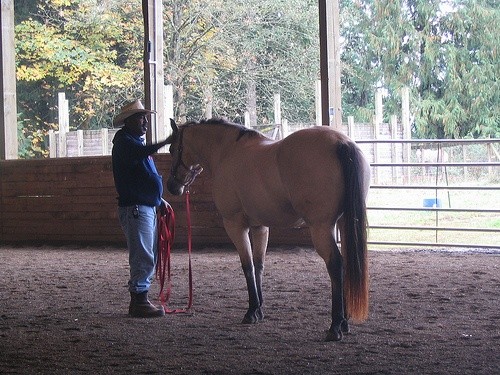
[128,291,166,317]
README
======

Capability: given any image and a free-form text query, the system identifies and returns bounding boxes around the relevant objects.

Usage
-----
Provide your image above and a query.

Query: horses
[165,116,371,342]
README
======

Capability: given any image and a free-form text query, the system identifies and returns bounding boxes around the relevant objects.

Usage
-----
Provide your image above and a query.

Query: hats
[114,99,157,128]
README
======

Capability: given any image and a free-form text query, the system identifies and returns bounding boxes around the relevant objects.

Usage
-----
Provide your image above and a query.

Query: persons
[111,101,177,317]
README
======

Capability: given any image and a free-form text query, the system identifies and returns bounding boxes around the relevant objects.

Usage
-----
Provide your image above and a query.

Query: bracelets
[161,142,165,146]
